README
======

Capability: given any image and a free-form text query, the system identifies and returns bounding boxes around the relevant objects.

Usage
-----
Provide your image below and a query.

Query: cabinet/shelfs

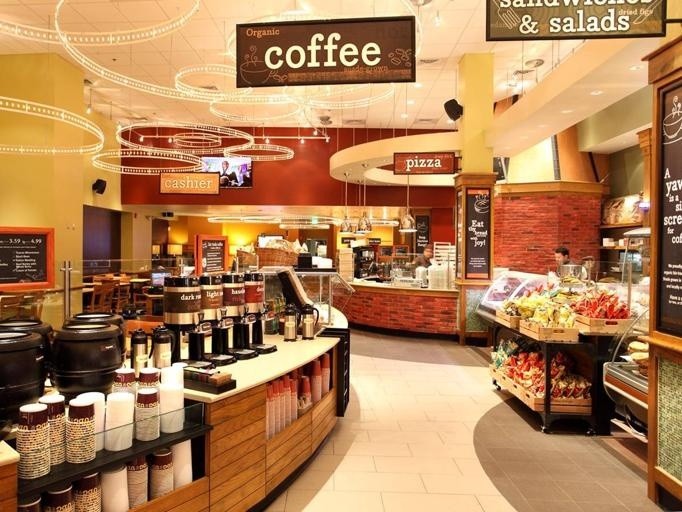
[600,225,643,286]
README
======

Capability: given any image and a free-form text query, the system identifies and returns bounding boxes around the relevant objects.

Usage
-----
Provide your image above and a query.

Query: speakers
[444,99,462,120]
[95,178,107,194]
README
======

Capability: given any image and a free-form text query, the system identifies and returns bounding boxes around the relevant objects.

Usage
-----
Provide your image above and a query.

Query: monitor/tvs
[199,156,253,190]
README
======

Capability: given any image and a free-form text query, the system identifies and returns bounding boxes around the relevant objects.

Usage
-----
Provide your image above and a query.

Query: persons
[216,159,238,187]
[408,245,433,277]
[552,247,576,275]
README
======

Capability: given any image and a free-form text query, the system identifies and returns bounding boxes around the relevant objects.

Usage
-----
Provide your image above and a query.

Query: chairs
[92,275,126,311]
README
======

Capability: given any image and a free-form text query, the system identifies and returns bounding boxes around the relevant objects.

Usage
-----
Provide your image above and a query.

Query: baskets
[236,247,299,269]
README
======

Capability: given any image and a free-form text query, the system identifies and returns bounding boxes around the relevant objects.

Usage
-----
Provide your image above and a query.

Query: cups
[16,395,96,479]
[112,367,161,443]
[265,354,330,440]
[17,472,102,512]
[131,326,176,377]
[127,449,174,509]
[284,303,319,341]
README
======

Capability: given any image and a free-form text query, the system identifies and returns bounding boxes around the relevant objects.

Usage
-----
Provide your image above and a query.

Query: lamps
[398,83,420,235]
[355,119,372,235]
[339,130,355,233]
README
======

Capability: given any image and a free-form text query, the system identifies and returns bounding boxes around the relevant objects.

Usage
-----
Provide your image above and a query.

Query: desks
[478,315,605,435]
